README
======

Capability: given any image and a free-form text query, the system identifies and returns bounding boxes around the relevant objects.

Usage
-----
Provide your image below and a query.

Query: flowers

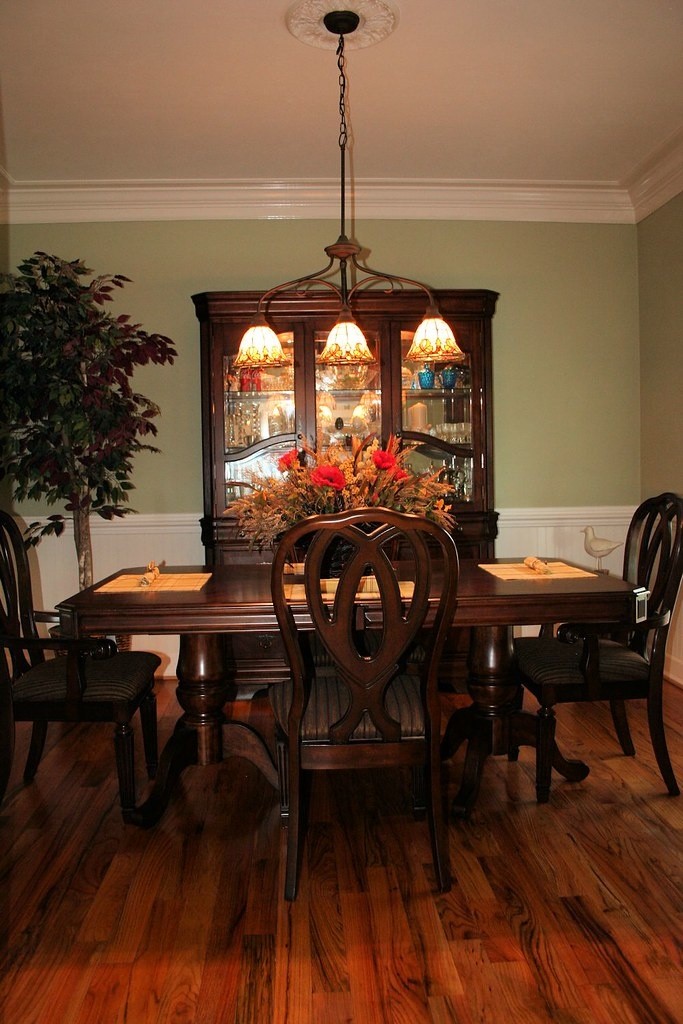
[224,428,464,555]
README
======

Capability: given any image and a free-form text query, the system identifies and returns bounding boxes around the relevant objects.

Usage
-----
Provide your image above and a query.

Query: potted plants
[0,248,181,660]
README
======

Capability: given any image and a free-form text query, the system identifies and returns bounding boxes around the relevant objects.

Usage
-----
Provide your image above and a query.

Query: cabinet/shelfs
[190,286,502,684]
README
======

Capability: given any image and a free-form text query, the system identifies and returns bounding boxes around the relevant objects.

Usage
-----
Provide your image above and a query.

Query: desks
[55,555,646,821]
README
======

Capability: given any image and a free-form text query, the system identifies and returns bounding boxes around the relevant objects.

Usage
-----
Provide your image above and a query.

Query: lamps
[231,0,466,371]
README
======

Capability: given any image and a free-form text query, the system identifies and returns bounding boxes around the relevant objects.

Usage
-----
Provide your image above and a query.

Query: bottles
[418,363,434,389]
[427,456,466,498]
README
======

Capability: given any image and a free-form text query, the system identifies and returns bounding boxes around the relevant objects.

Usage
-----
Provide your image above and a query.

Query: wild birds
[579,526,625,572]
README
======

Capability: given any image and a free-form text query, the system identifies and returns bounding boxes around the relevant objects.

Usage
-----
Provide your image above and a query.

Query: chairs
[0,509,163,826]
[266,504,460,902]
[501,492,683,807]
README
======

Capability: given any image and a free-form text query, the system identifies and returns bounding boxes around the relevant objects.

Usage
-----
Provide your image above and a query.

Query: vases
[300,536,374,579]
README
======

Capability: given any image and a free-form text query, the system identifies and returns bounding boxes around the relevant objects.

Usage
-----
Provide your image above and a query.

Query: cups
[435,422,472,444]
[438,369,456,388]
